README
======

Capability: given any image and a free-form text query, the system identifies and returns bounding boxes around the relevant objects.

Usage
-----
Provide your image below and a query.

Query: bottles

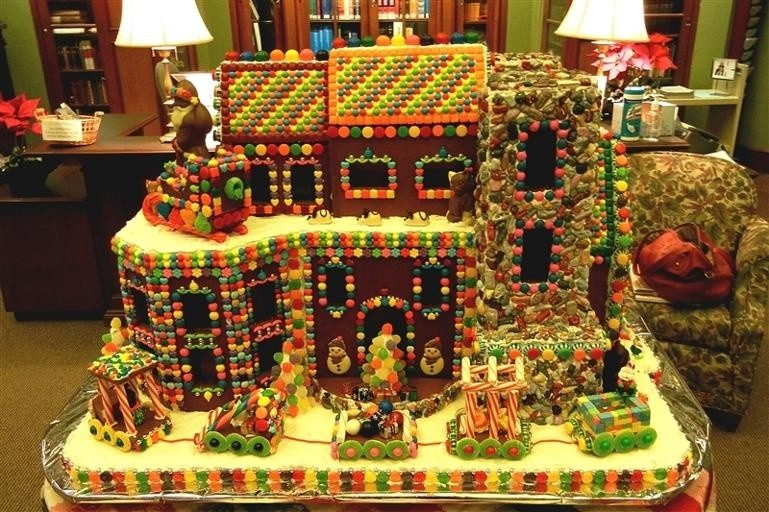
[620,86,643,141]
[70,76,108,106]
[58,38,97,70]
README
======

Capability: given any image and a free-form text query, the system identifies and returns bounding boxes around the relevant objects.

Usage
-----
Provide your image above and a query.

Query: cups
[641,111,661,143]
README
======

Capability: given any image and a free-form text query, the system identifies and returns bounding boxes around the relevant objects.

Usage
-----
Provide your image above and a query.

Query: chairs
[614,151,769,434]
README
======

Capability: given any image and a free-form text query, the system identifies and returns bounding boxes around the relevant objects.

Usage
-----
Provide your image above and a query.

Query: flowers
[585,30,681,86]
[0,92,49,145]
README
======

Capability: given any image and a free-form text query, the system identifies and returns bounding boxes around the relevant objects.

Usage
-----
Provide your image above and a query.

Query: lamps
[553,1,650,109]
[112,1,215,132]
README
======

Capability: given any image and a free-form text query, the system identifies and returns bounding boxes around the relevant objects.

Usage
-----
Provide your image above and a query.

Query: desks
[623,135,691,155]
[653,60,749,159]
[0,110,215,325]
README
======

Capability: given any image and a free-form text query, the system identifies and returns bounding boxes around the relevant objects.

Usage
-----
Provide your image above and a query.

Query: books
[49,10,108,105]
[308,0,429,53]
[250,0,273,52]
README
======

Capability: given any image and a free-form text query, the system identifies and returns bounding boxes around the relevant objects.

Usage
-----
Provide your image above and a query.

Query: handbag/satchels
[632,221,735,305]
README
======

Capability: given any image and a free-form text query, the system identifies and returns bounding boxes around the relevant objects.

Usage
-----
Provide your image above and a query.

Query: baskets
[32,106,105,147]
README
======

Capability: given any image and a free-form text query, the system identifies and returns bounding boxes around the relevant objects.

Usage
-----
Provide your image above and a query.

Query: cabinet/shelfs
[228,0,510,64]
[558,1,692,88]
[27,0,203,141]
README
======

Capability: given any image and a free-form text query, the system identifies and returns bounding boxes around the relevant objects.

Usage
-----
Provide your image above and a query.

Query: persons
[447,173,473,222]
[171,80,212,166]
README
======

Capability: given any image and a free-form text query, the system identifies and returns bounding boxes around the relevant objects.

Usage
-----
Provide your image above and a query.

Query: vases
[8,156,54,200]
